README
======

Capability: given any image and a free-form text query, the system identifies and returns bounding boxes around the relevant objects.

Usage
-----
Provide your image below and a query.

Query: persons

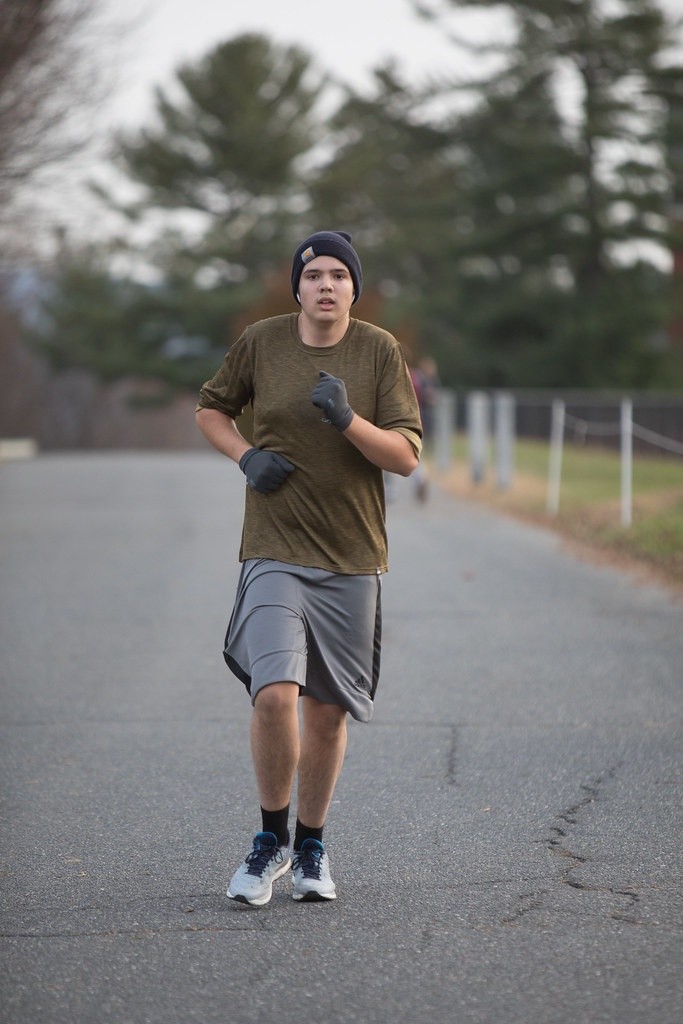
[381,348,440,504]
[195,231,423,905]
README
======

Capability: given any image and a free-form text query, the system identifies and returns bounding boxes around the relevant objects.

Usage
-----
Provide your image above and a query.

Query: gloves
[238,447,297,495]
[311,371,354,432]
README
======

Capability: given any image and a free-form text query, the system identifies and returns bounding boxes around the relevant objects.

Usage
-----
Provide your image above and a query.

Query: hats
[291,230,362,306]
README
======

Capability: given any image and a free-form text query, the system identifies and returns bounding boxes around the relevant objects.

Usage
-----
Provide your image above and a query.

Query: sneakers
[291,838,336,901]
[226,832,292,905]
